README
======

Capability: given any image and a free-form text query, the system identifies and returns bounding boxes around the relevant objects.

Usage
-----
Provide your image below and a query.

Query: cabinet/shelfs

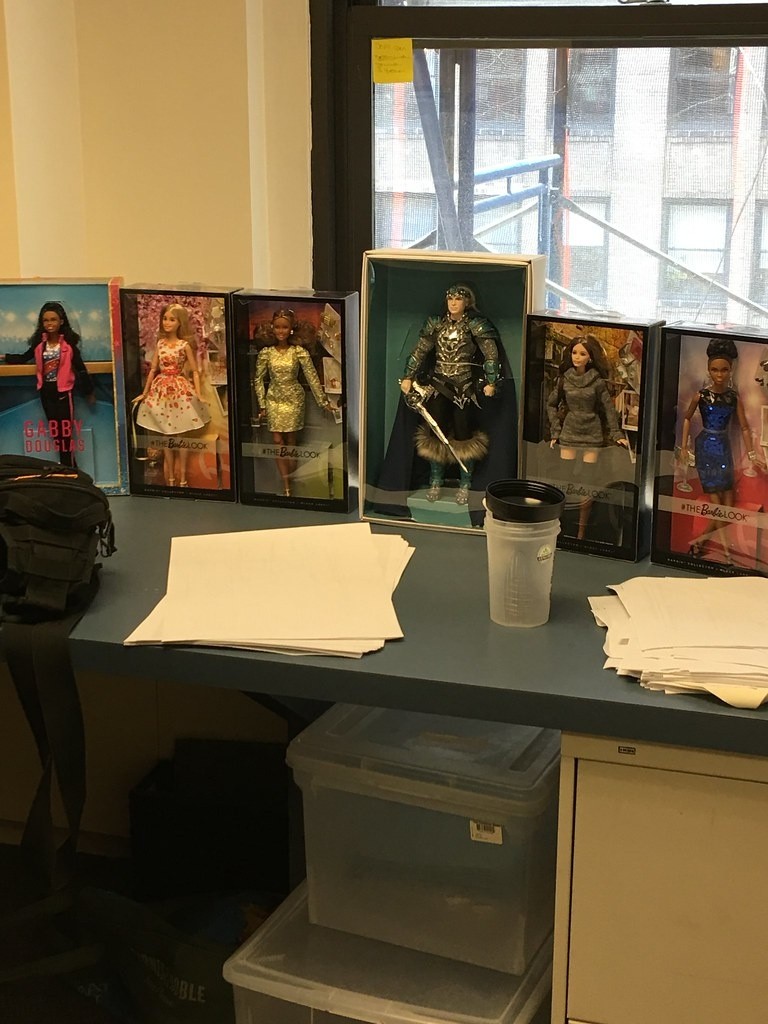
[550,731,768,1024]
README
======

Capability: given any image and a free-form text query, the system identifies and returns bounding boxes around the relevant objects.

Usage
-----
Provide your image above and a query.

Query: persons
[254,310,339,497]
[547,336,630,541]
[401,287,499,505]
[131,304,210,487]
[679,339,767,564]
[0,301,96,467]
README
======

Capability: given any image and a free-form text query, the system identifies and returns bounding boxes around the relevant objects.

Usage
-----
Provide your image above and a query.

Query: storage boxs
[222,878,554,1024]
[358,250,547,536]
[285,701,559,976]
[231,288,359,514]
[118,283,243,503]
[520,309,667,564]
[651,320,768,577]
[0,277,131,496]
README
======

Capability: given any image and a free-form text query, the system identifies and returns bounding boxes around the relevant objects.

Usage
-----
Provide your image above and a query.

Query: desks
[0,493,768,839]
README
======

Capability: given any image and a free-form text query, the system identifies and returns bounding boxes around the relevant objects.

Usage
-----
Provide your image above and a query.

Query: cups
[482,478,565,627]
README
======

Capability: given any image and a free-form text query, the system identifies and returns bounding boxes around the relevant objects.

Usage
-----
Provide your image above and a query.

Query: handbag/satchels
[0,454,112,621]
